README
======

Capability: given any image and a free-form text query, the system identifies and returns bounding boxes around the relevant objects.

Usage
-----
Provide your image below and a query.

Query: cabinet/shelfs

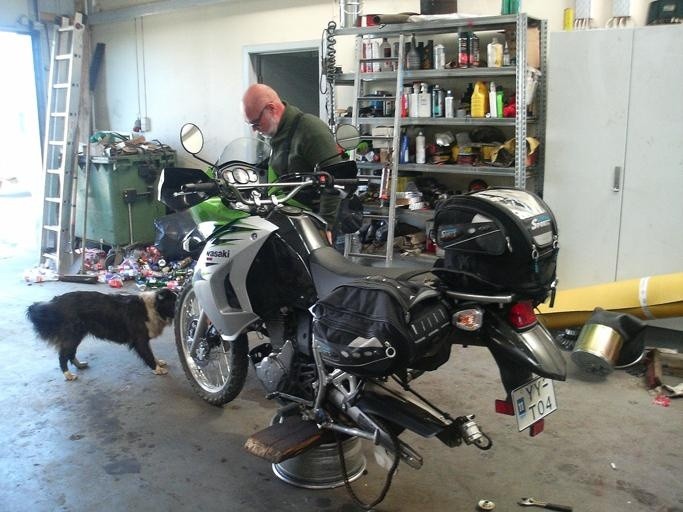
[322,12,544,282]
[541,27,683,330]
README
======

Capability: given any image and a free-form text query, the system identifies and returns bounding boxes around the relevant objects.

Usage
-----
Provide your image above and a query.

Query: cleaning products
[400,132,409,163]
[416,131,426,163]
[379,36,392,71]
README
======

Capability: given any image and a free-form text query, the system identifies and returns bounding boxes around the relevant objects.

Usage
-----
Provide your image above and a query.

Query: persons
[241,84,342,245]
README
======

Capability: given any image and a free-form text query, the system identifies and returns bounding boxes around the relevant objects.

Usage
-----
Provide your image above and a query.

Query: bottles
[563,8,574,32]
[79,240,199,292]
[377,31,421,73]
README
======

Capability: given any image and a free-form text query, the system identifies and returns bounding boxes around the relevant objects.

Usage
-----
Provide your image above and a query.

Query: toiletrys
[407,31,420,70]
[486,37,503,67]
[488,80,505,118]
[408,80,455,118]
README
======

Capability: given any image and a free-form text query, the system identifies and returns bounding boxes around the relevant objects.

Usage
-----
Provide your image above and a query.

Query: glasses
[242,100,273,128]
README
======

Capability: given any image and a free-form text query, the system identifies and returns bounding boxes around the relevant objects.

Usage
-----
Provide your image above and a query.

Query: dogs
[25,287,193,381]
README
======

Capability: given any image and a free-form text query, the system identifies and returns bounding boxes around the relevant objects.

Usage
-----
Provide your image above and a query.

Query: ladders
[343,35,405,269]
[37,12,86,272]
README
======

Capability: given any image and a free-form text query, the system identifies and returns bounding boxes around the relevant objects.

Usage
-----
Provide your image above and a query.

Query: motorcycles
[174,125,567,506]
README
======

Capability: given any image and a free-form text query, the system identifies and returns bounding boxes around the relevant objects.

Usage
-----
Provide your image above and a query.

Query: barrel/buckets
[471,80,487,117]
[572,322,624,376]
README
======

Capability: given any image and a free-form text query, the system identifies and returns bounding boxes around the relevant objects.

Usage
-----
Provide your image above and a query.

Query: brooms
[58,102,99,283]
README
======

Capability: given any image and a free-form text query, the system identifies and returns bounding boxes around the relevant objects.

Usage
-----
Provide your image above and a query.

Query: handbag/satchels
[310,271,454,380]
